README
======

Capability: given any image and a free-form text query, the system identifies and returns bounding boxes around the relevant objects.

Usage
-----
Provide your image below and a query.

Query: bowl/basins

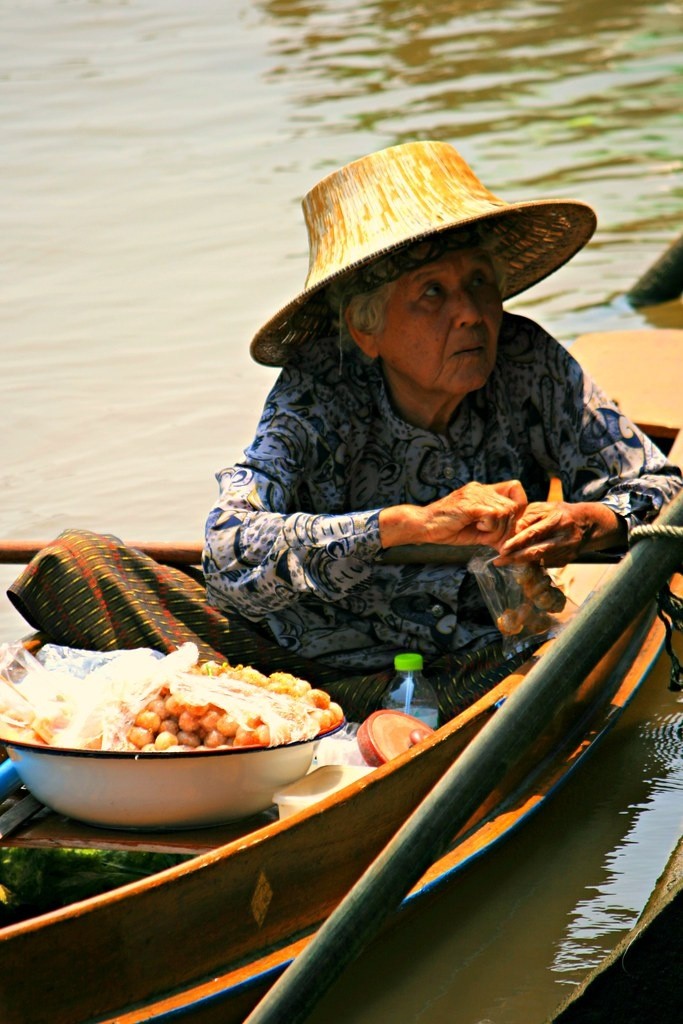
[0,718,347,833]
[272,765,377,820]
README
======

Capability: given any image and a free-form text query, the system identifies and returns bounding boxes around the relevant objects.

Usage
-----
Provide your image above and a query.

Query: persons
[8,141,683,723]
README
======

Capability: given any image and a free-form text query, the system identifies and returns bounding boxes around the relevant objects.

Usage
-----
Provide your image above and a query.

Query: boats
[0,327,683,1024]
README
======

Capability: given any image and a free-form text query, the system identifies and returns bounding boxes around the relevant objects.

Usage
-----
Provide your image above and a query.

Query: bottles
[384,654,439,730]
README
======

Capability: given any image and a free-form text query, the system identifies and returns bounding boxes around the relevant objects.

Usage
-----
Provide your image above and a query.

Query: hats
[250,141,597,365]
